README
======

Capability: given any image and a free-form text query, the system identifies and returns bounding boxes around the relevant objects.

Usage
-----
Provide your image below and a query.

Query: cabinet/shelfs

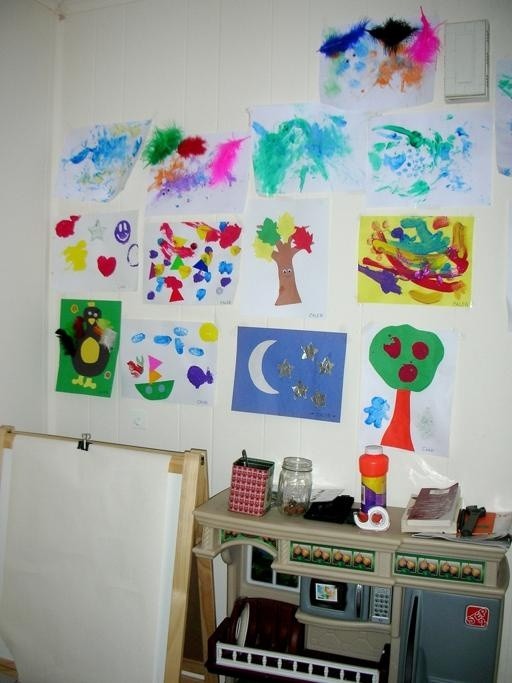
[191,487,512,682]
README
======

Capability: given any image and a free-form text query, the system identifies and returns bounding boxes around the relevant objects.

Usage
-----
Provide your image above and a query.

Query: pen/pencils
[242,449,247,468]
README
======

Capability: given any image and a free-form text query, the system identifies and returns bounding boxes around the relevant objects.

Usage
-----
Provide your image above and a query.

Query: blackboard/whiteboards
[0,424,201,683]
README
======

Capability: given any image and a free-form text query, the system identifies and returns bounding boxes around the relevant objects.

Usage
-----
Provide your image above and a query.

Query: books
[401,494,463,534]
[457,512,497,535]
[406,482,461,528]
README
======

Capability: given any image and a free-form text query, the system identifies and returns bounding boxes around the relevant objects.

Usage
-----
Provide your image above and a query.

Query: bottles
[276,458,313,517]
[359,446,388,514]
